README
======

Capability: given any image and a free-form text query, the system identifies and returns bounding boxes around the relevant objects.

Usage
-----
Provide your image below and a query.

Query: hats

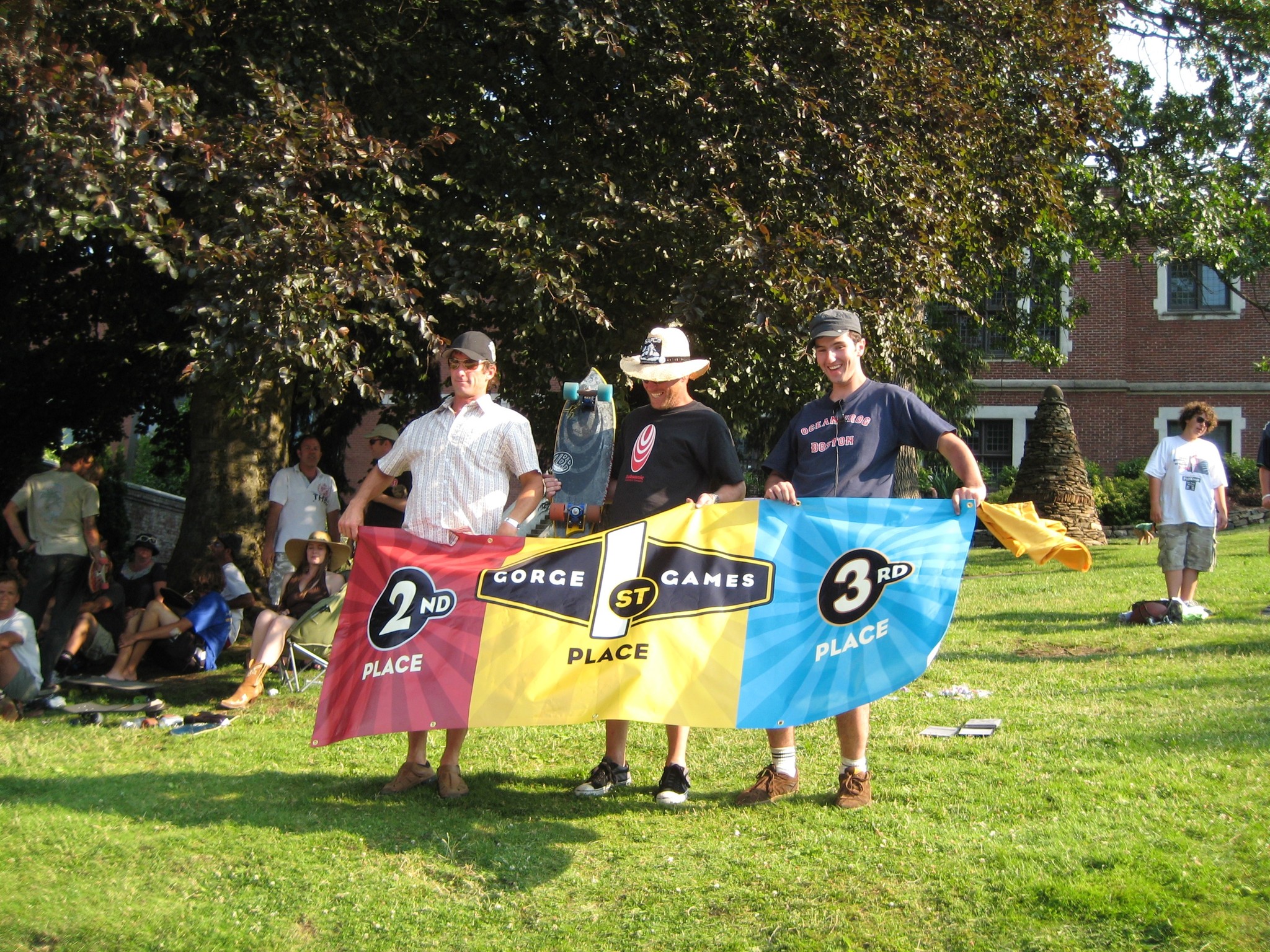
[620,327,711,381]
[441,331,496,363]
[808,309,862,348]
[363,423,399,441]
[129,534,160,556]
[218,533,242,551]
[285,531,350,572]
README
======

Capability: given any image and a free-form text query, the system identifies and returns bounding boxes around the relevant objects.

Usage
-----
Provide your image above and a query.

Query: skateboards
[57,697,173,728]
[67,675,164,701]
[88,555,115,593]
[550,367,616,539]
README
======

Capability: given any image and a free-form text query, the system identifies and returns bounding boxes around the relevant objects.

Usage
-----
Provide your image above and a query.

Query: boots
[219,653,269,708]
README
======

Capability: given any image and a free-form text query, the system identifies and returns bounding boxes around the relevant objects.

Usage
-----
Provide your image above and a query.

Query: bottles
[120,719,146,728]
[142,718,158,728]
[158,715,184,728]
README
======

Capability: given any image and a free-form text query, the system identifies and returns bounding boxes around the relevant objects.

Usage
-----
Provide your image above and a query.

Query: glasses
[643,380,664,384]
[833,399,845,424]
[136,535,157,544]
[1193,415,1212,427]
[448,358,491,370]
[214,542,226,549]
[369,439,385,445]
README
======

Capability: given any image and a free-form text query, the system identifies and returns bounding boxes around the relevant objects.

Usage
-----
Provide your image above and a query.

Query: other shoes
[1185,600,1208,619]
[37,684,60,696]
[170,722,219,736]
[380,760,435,794]
[183,711,240,722]
[437,765,470,797]
[1262,604,1270,615]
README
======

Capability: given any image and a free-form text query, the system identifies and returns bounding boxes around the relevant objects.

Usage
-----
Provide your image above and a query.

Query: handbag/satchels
[1129,599,1174,625]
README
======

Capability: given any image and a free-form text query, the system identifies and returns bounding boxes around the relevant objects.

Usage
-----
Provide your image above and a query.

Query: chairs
[277,570,358,695]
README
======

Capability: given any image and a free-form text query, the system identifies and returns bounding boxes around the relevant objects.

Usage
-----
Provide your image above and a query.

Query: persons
[734,309,986,808]
[1255,422,1270,615]
[220,531,351,709]
[340,423,412,528]
[0,444,255,720]
[259,434,341,671]
[1133,522,1158,545]
[543,328,746,804]
[922,475,937,498]
[338,332,547,798]
[1143,401,1229,617]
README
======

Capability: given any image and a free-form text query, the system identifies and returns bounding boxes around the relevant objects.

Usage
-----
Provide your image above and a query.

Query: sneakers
[575,756,632,796]
[656,762,691,804]
[735,765,799,805]
[834,767,872,809]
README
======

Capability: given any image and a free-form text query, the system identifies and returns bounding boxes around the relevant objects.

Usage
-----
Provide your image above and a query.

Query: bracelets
[1262,494,1270,500]
[504,517,520,530]
[713,493,719,503]
